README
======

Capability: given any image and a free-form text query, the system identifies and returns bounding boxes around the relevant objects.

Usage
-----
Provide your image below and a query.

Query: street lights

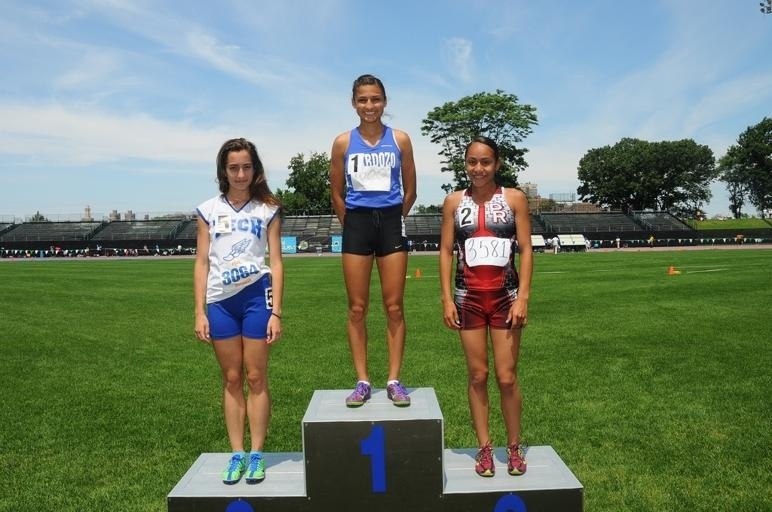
[697,210,701,221]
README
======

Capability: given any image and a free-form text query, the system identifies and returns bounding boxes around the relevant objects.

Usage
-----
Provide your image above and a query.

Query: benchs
[0,212,701,241]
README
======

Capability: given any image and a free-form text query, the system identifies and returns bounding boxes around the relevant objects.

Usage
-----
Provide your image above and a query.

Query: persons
[736,233,744,248]
[615,236,621,249]
[423,239,428,251]
[551,236,559,254]
[190,138,290,486]
[328,74,419,408]
[438,133,536,480]
[649,235,654,248]
[178,243,183,254]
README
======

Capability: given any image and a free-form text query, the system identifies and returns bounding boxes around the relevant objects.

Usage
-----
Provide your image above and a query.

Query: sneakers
[505,440,528,475]
[346,381,371,406]
[476,441,494,477]
[388,381,409,405]
[222,451,246,484]
[246,452,266,481]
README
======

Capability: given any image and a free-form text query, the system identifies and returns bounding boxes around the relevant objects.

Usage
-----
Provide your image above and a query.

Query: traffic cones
[667,265,675,275]
[414,267,422,278]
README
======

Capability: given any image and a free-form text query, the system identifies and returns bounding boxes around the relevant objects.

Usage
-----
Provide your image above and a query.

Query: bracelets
[271,312,283,320]
